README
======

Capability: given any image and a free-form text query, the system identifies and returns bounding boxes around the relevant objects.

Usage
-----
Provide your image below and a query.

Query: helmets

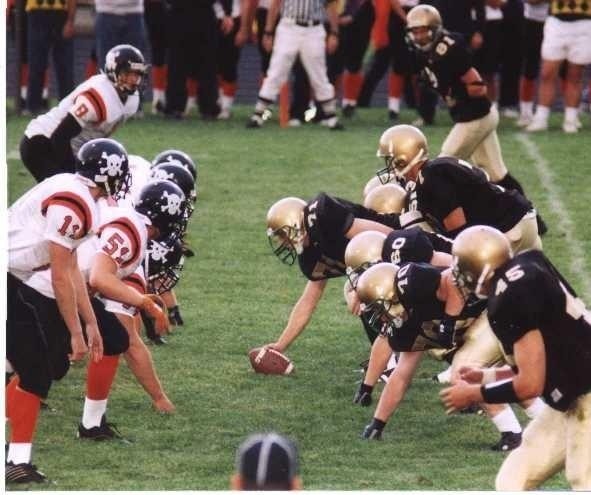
[266,197,309,266]
[148,162,198,203]
[136,181,194,248]
[147,230,194,295]
[104,44,152,96]
[76,137,132,201]
[357,262,409,338]
[362,187,407,214]
[404,5,443,54]
[451,225,513,309]
[148,151,197,182]
[378,124,430,184]
[344,230,388,293]
[363,173,397,199]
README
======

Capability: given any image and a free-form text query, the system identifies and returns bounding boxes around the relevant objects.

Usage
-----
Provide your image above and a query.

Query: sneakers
[561,117,579,134]
[490,432,522,452]
[527,116,550,135]
[312,97,345,132]
[5,461,55,485]
[518,112,534,127]
[247,96,276,130]
[77,413,134,446]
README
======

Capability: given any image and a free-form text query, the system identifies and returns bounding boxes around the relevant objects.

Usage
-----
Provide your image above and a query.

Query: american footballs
[248,347,295,377]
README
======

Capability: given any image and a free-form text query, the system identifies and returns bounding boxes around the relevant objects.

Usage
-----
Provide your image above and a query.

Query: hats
[237,434,298,491]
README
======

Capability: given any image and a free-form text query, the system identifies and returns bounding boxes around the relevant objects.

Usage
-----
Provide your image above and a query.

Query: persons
[517,5,544,130]
[473,3,520,117]
[91,0,158,119]
[7,136,133,483]
[4,179,189,446]
[362,122,547,254]
[152,0,234,121]
[441,226,590,493]
[19,42,151,184]
[229,433,306,490]
[124,142,199,206]
[524,0,590,135]
[402,3,549,237]
[336,0,404,123]
[248,193,460,384]
[355,263,550,453]
[94,161,196,347]
[363,183,462,245]
[341,231,458,312]
[246,0,346,132]
[20,0,80,121]
